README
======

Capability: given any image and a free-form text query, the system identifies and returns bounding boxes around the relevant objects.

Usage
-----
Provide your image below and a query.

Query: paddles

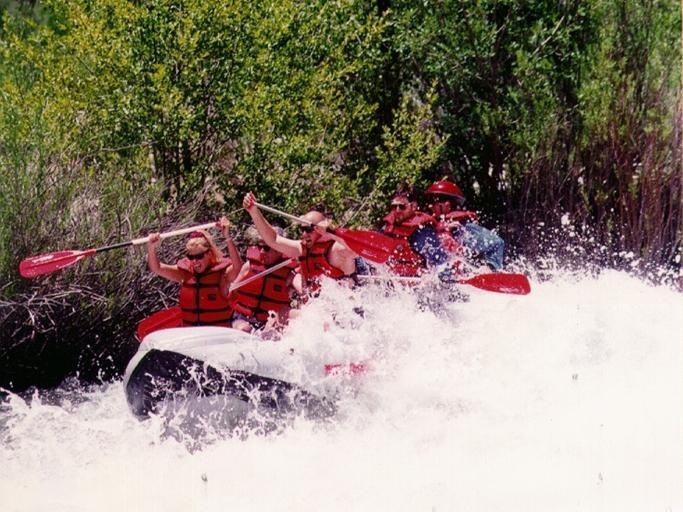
[356,273,530,295]
[19,221,221,279]
[137,256,297,341]
[247,199,396,263]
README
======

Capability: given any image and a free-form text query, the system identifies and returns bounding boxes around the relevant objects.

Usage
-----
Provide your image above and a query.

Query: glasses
[429,195,452,204]
[257,243,271,252]
[298,225,313,233]
[391,204,406,210]
[186,248,210,259]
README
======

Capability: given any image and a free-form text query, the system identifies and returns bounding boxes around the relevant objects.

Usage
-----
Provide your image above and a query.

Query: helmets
[424,181,464,199]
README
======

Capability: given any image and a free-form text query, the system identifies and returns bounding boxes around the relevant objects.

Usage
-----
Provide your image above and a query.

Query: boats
[119,326,335,428]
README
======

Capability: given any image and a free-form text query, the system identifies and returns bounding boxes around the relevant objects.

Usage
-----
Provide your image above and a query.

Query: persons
[424,179,504,275]
[241,190,360,317]
[145,216,240,329]
[376,190,467,281]
[224,225,304,340]
